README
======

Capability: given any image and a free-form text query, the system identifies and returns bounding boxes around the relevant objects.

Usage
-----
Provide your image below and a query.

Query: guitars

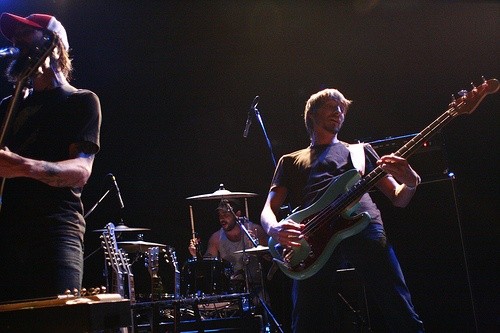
[1,33,61,194]
[99,232,206,333]
[268,74,500,280]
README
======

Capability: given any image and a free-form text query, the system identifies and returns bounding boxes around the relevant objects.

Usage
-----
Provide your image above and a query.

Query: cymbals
[235,246,271,254]
[116,241,167,247]
[185,189,256,199]
[93,224,151,231]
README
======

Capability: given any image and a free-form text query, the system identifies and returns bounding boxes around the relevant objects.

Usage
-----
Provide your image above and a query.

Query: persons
[0,13,102,301]
[261,88,424,333]
[188,199,278,333]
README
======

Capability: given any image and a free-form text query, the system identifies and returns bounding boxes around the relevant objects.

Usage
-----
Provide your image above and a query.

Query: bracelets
[406,186,417,190]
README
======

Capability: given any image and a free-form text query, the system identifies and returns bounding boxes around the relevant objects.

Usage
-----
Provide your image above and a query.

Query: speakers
[331,177,480,333]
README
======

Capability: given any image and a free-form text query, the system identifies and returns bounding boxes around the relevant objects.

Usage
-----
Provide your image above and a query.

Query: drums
[185,258,237,312]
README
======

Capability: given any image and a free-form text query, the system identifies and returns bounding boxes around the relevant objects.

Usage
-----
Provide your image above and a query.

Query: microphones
[0,46,20,57]
[111,175,125,209]
[242,98,259,138]
